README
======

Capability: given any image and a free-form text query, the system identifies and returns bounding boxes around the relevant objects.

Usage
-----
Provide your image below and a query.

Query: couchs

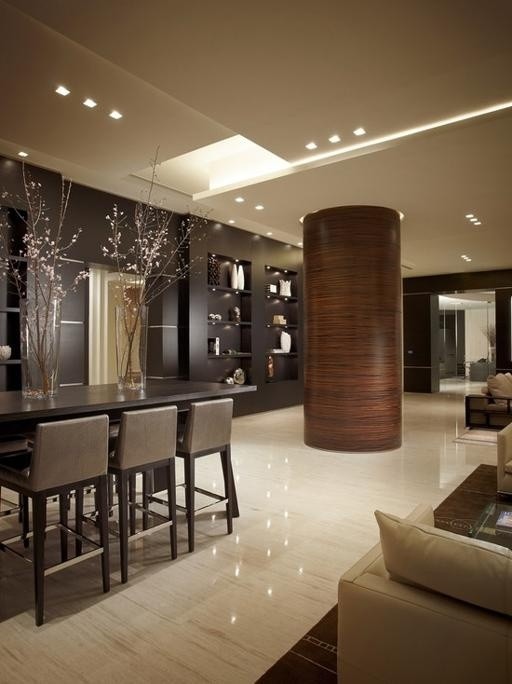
[466,372,512,430]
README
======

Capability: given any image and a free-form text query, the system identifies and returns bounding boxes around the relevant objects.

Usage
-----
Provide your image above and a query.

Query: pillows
[372,505,511,612]
[486,372,512,405]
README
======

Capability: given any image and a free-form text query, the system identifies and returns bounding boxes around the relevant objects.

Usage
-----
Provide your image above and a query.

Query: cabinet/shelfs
[180,213,303,419]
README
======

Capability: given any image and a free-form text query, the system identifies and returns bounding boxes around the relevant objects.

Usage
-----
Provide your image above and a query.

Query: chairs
[0,396,235,627]
[335,504,511,683]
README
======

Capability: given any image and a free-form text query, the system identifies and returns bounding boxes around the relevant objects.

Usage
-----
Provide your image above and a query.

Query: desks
[0,379,257,518]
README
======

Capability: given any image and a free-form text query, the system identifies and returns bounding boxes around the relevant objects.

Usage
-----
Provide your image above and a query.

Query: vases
[17,294,60,400]
[113,304,149,390]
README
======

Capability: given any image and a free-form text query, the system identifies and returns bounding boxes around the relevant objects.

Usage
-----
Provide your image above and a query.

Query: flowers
[102,143,213,324]
[1,156,95,389]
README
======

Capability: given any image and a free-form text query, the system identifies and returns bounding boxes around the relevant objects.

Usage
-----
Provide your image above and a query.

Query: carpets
[255,464,510,682]
